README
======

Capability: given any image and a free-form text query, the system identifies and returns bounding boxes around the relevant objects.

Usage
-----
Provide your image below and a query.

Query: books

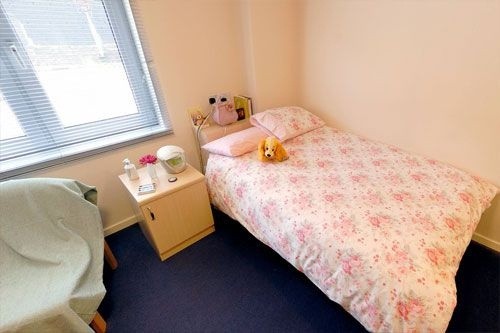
[233,94,253,118]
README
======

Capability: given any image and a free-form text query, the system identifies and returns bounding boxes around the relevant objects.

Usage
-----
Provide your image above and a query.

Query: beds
[204,127,500,332]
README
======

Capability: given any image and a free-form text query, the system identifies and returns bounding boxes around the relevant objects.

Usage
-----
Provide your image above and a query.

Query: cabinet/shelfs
[189,97,254,176]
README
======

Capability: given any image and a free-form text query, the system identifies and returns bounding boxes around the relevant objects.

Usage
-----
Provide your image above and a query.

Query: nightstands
[118,161,215,263]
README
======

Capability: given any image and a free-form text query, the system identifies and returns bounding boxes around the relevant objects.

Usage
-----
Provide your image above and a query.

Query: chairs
[0,178,119,333]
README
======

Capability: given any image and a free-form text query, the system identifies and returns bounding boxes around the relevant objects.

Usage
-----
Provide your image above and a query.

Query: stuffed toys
[257,135,289,164]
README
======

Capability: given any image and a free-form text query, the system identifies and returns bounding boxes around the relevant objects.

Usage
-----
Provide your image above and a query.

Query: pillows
[200,127,268,158]
[249,106,325,142]
